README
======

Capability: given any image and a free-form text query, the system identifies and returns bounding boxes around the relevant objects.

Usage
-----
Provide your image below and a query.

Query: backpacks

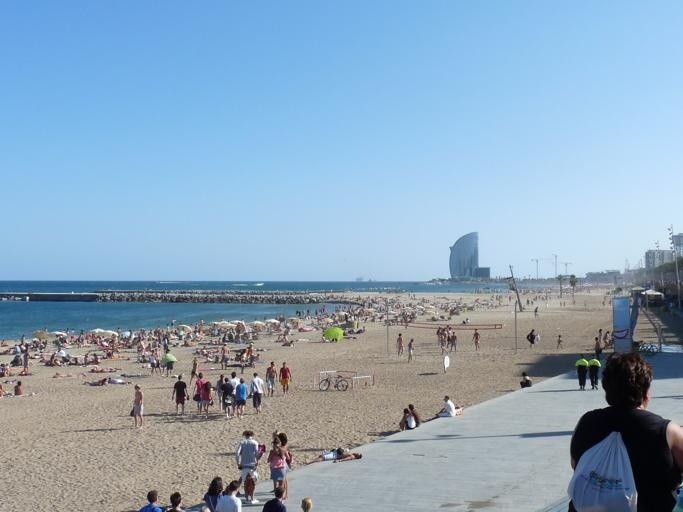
[567,430,640,512]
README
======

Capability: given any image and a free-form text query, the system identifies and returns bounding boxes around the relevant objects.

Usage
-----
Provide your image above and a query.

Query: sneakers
[130,409,265,430]
[243,498,260,505]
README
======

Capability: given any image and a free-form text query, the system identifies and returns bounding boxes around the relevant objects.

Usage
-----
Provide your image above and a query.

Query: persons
[103,323,174,377]
[138,430,312,511]
[171,361,293,420]
[132,383,145,429]
[475,288,575,317]
[363,293,468,328]
[173,319,257,374]
[399,395,456,430]
[396,324,482,363]
[519,328,614,388]
[267,305,366,348]
[568,350,683,512]
[1,328,100,394]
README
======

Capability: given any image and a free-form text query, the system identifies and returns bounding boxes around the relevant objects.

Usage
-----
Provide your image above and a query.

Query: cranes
[530,257,553,278]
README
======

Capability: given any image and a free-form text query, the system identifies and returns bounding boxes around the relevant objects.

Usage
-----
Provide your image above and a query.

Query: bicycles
[317,371,351,391]
[637,339,659,356]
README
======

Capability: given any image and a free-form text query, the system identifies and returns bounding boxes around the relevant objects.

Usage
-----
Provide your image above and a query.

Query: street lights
[667,223,682,311]
[653,240,664,293]
